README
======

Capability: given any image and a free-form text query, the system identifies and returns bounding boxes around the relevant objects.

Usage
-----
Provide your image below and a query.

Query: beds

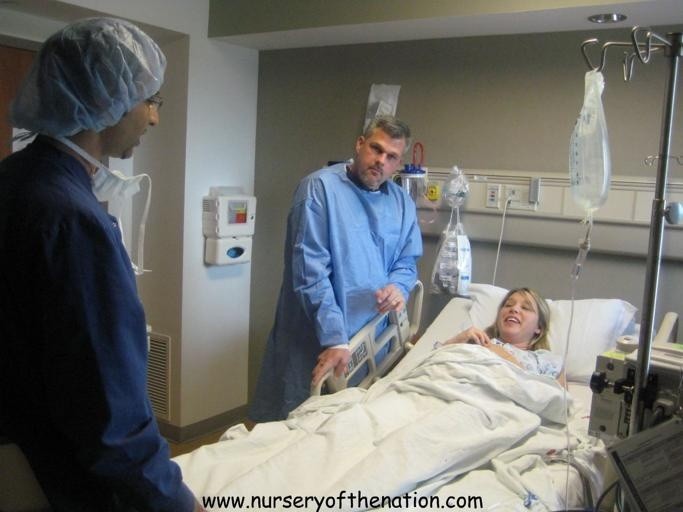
[120,275,635,511]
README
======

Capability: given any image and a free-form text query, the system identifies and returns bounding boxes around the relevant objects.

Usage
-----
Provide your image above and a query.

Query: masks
[89,167,142,204]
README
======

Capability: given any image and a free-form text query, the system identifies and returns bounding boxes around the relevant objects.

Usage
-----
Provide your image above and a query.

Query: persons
[0,16,209,510]
[245,115,422,429]
[436,285,570,393]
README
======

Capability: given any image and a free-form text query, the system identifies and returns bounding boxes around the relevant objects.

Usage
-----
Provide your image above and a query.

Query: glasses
[145,97,163,111]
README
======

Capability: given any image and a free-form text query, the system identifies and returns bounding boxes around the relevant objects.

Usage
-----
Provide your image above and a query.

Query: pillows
[457,279,636,387]
